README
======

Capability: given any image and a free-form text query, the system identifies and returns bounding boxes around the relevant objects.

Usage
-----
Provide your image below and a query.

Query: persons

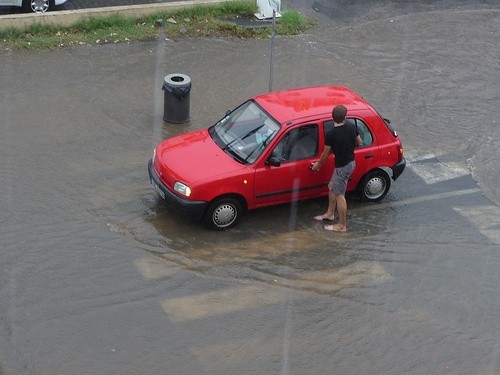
[310,105,362,232]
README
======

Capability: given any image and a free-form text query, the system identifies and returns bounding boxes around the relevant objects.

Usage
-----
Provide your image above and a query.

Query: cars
[147,84,406,231]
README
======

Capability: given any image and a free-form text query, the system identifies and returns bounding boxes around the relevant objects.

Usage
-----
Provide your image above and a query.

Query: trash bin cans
[162,73,193,125]
